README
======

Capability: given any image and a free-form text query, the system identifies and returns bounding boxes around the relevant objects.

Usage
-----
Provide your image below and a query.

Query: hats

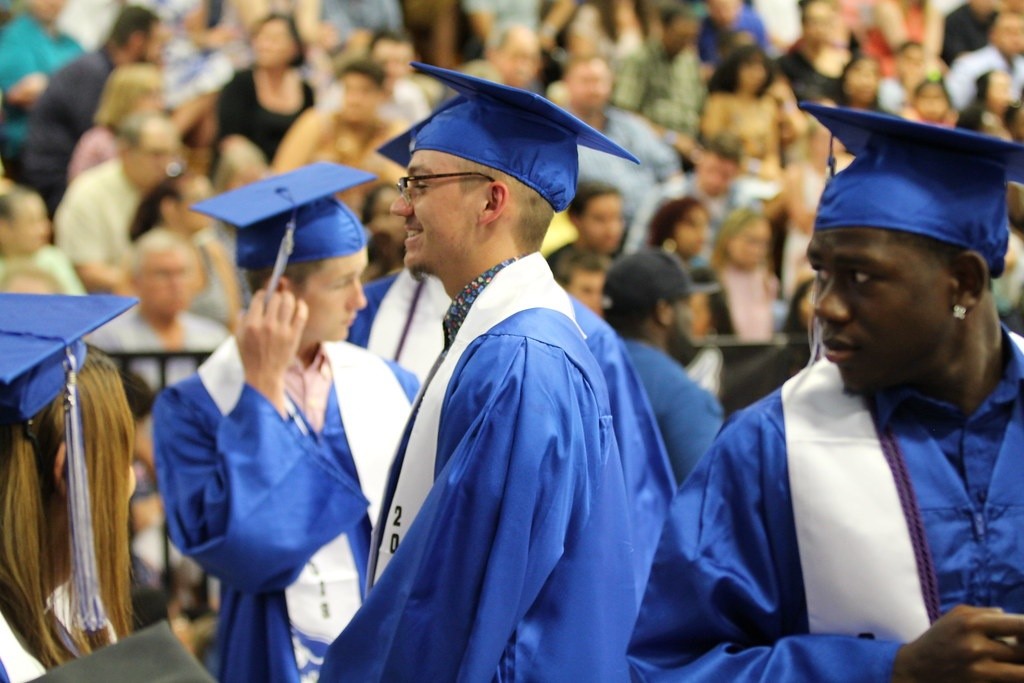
[186,158,378,373]
[780,98,1024,400]
[374,92,467,168]
[410,55,640,215]
[1,285,142,631]
[602,250,723,304]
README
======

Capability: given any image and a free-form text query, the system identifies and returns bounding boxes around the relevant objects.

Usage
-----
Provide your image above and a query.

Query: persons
[626,103,1024,683]
[0,295,143,683]
[317,64,639,683]
[153,162,420,683]
[0,0,1024,656]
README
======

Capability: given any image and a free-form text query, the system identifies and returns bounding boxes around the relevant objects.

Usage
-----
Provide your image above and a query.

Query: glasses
[398,171,497,197]
